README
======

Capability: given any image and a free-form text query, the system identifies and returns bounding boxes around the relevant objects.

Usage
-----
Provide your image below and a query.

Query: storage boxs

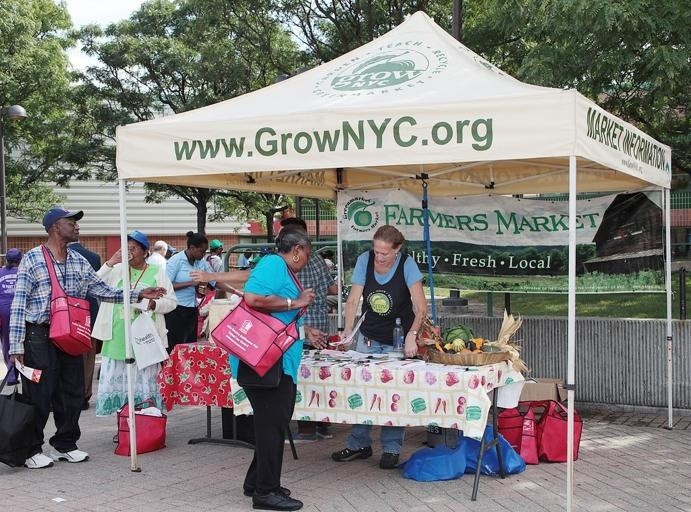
[516,378,569,405]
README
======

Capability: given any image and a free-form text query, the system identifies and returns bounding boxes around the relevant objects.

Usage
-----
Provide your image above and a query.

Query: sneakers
[315,430,333,439]
[332,446,373,462]
[51,447,90,463]
[284,433,318,444]
[380,453,399,469]
[24,453,54,468]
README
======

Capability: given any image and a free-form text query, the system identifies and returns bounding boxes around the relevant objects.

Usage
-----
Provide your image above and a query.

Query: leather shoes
[244,487,291,496]
[253,490,303,511]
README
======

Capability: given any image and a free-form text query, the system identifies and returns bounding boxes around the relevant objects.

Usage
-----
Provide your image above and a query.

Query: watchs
[408,330,418,335]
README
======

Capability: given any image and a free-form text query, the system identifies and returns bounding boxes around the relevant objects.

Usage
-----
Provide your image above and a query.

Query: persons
[188,216,338,444]
[206,239,226,299]
[323,250,335,272]
[236,224,327,511]
[331,225,427,469]
[0,248,24,386]
[238,249,252,270]
[66,238,101,411]
[90,230,178,443]
[164,231,245,357]
[8,208,167,469]
[146,239,168,265]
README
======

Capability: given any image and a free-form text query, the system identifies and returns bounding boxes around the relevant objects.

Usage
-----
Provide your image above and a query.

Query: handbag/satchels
[130,298,170,369]
[537,400,583,463]
[497,400,540,465]
[0,360,40,467]
[237,358,282,387]
[115,398,167,456]
[211,296,299,379]
[49,295,93,356]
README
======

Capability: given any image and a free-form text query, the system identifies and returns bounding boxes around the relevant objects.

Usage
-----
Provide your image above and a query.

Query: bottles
[392,318,404,352]
[198,268,207,295]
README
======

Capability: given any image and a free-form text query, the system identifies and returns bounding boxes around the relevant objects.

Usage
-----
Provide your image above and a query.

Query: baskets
[428,348,509,365]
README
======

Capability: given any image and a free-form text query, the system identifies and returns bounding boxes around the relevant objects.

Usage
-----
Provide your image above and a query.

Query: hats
[127,230,149,250]
[209,239,224,249]
[43,208,83,232]
[6,248,22,261]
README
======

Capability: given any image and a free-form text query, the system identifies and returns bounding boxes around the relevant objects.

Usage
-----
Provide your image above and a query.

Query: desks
[231,348,526,501]
[156,341,256,449]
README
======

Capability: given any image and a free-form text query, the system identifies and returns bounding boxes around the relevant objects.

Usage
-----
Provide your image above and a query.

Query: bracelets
[232,288,235,294]
[287,298,292,311]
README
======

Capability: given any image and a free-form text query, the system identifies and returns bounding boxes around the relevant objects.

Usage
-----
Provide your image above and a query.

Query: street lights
[0,103,25,266]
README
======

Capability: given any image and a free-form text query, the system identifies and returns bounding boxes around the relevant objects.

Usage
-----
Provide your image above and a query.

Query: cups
[128,253,133,261]
